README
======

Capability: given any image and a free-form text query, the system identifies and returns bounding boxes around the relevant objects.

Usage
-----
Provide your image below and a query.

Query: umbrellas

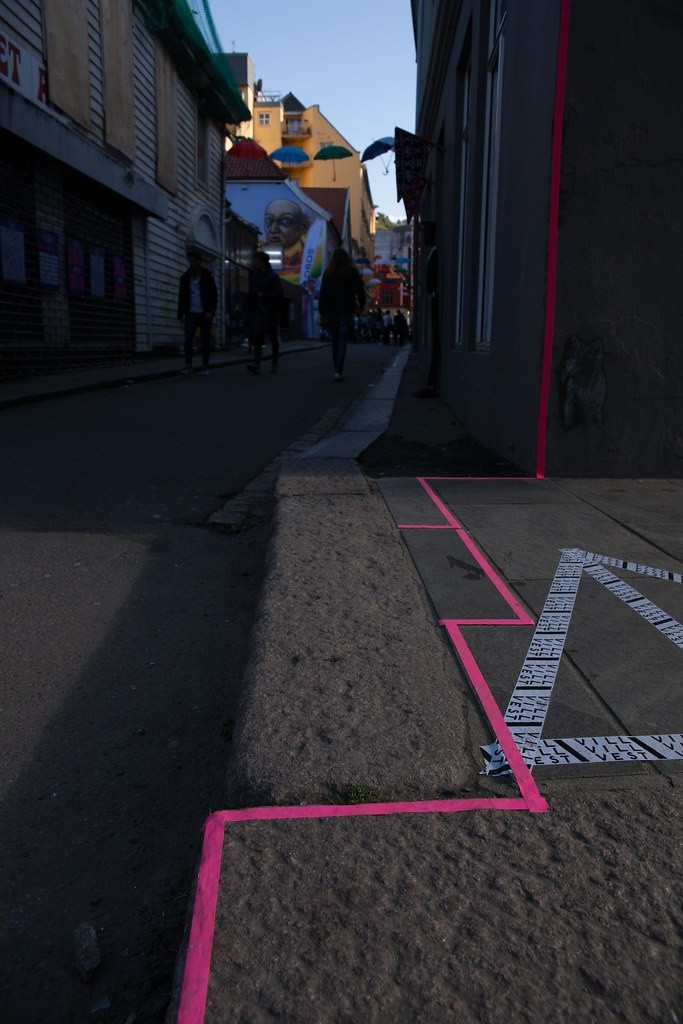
[361,136,394,175]
[226,138,268,172]
[314,143,353,181]
[272,144,309,181]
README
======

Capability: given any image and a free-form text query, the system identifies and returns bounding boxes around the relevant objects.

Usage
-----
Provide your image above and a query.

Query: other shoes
[200,368,210,375]
[271,366,278,373]
[333,372,342,380]
[180,369,194,375]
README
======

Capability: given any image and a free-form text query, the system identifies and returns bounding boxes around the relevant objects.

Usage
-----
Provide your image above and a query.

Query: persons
[413,220,440,402]
[318,249,366,381]
[178,246,219,377]
[352,307,409,346]
[243,251,284,375]
